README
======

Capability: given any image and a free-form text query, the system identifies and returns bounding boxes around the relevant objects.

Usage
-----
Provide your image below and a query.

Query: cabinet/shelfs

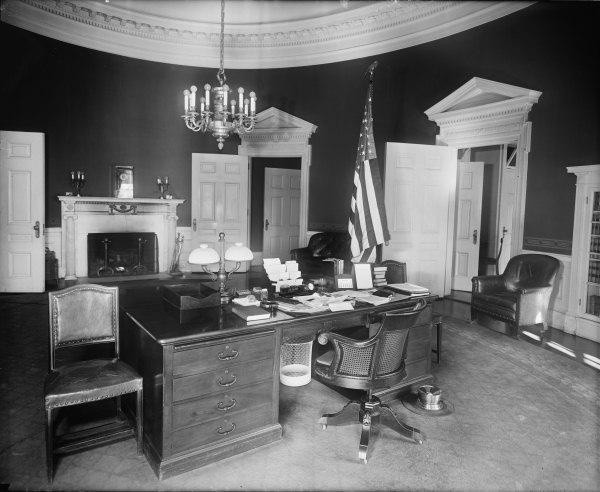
[567,164,600,343]
[57,196,184,278]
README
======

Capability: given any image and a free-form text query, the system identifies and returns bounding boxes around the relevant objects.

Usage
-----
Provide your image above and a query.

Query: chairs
[316,296,427,464]
[371,259,406,283]
[42,283,145,485]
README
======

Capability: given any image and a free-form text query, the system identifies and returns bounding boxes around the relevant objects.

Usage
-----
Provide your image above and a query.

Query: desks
[118,282,440,482]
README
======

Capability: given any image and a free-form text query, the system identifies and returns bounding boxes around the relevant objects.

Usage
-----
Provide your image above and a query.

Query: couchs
[289,232,352,278]
[470,253,566,338]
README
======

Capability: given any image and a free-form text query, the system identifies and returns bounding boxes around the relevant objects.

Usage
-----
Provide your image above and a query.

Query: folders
[387,282,428,296]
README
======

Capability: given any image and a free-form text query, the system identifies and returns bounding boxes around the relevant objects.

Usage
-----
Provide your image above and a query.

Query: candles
[71,171,86,182]
[157,176,168,185]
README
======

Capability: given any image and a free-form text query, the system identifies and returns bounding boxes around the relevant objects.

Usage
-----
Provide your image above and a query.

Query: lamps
[187,233,254,303]
[182,2,257,150]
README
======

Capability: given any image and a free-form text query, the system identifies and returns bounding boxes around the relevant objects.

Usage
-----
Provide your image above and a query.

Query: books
[371,264,388,288]
[231,305,271,322]
[387,282,430,297]
[323,258,344,276]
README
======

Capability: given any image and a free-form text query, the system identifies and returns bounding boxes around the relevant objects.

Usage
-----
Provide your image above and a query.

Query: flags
[347,75,390,262]
